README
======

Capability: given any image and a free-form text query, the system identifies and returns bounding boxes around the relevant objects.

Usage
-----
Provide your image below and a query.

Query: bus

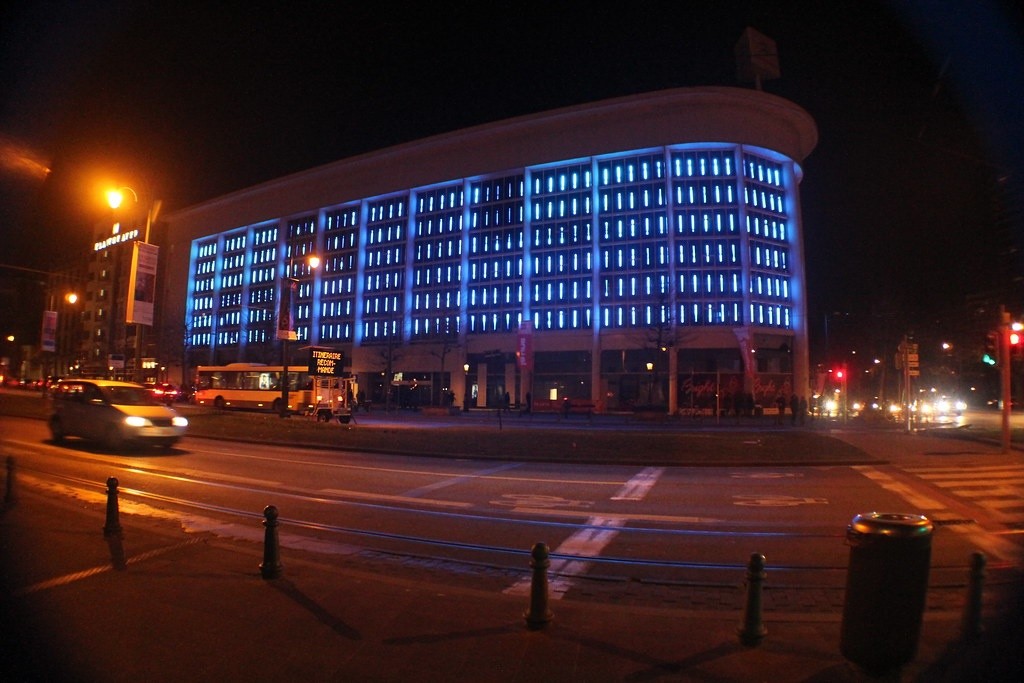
[195,363,359,415]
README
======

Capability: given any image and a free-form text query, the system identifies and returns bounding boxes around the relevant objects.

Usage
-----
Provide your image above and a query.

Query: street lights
[108,186,143,383]
[280,233,321,420]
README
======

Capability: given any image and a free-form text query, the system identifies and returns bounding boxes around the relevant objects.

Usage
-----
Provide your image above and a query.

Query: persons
[712,383,756,424]
[438,389,573,419]
[778,391,826,428]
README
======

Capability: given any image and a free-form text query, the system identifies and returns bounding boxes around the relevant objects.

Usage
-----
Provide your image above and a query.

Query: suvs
[48,379,189,452]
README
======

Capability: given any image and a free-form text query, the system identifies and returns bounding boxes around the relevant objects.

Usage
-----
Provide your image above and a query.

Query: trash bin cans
[840,512,934,668]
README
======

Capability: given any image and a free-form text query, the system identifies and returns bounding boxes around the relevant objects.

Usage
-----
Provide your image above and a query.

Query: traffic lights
[1010,331,1022,349]
[834,364,845,382]
[980,354,997,370]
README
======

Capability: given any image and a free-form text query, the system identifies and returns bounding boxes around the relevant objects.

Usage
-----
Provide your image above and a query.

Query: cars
[141,383,179,402]
[820,390,967,416]
